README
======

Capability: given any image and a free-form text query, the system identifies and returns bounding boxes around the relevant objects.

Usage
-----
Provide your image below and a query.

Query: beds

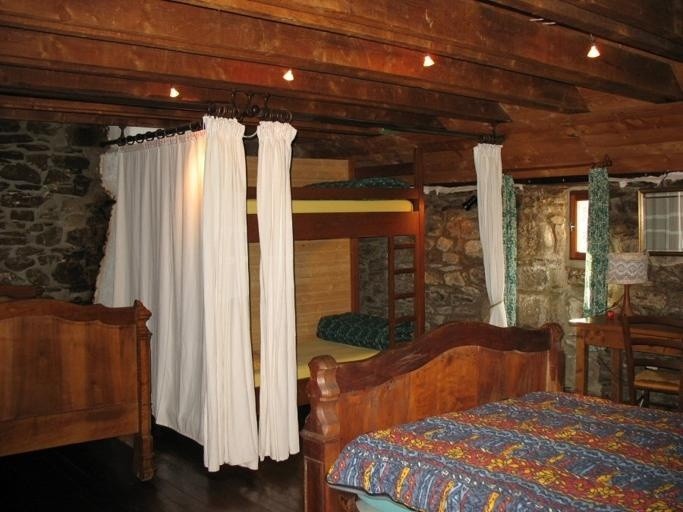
[247,184,424,416]
[0,284,154,481]
[300,321,683,512]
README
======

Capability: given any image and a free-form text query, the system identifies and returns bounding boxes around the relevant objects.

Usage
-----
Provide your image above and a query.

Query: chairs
[618,316,683,411]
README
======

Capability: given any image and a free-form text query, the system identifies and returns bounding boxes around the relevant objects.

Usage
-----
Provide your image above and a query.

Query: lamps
[607,252,649,320]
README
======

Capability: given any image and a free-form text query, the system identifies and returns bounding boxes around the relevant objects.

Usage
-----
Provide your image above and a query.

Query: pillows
[316,312,414,351]
[303,176,410,190]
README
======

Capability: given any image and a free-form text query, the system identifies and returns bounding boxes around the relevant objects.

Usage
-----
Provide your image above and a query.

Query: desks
[569,313,683,411]
